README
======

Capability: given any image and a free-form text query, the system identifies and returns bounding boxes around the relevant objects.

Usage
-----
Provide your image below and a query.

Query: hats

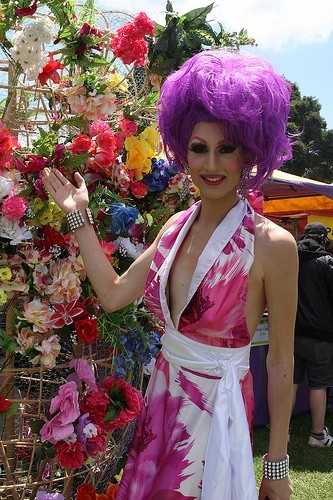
[303,222,330,239]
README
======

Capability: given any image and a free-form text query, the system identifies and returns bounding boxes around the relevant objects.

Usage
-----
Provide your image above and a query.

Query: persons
[43,49,299,500]
[286,221,333,447]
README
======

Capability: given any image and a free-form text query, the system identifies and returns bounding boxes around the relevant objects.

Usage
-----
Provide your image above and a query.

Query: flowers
[0,0,258,500]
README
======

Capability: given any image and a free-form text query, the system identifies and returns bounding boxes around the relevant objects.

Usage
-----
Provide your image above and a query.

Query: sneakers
[306,426,333,447]
[287,426,292,442]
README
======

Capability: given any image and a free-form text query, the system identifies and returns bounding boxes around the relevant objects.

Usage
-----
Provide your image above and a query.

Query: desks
[250,312,310,428]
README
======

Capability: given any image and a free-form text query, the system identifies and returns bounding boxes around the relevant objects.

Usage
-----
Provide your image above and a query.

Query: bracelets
[66,207,94,231]
[262,453,290,480]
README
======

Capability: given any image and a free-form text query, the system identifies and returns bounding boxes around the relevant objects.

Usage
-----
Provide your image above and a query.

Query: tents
[248,163,333,239]
